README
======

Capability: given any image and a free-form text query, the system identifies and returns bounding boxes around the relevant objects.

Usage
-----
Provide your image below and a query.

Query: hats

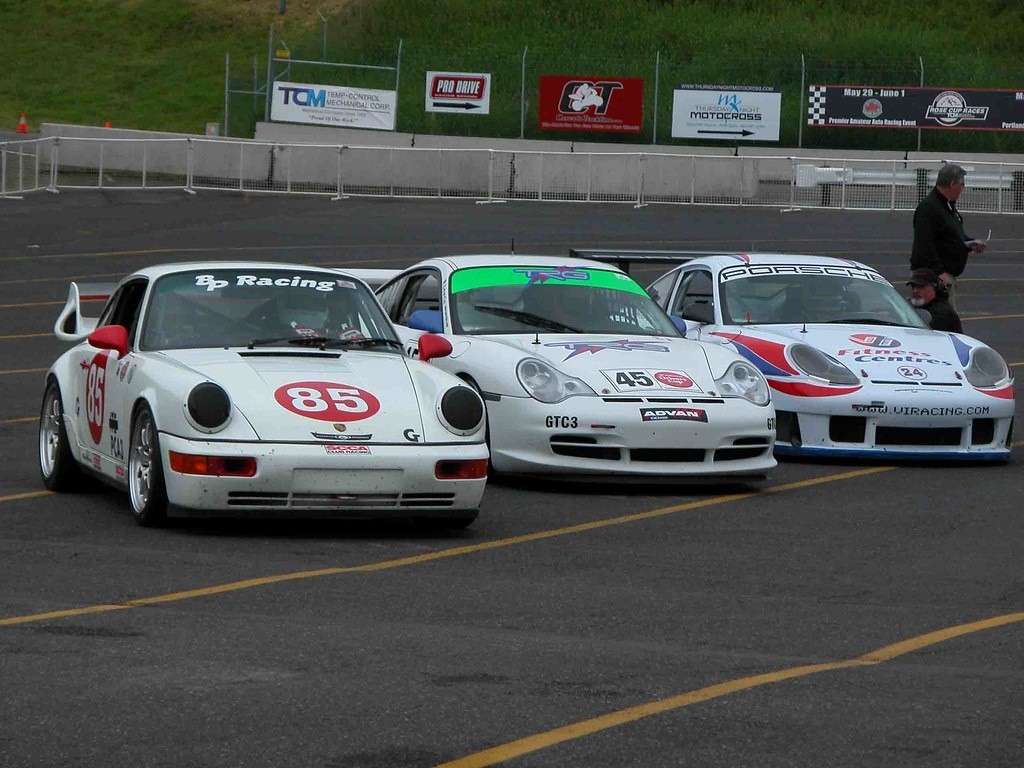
[906,268,939,287]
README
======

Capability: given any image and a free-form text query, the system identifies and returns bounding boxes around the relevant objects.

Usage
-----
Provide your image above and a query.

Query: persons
[552,286,647,334]
[905,164,989,336]
[278,284,368,343]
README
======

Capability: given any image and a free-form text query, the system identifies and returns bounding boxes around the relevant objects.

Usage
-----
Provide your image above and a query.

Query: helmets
[550,287,589,329]
[268,286,330,329]
[799,279,847,320]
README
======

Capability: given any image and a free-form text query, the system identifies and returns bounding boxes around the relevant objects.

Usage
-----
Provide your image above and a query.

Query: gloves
[290,325,323,340]
[338,326,367,343]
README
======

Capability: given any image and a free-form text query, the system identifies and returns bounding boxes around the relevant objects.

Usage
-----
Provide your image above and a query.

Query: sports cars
[37,261,489,535]
[328,255,778,492]
[570,246,1015,467]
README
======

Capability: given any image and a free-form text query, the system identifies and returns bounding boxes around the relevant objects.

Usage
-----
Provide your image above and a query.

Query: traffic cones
[15,113,29,134]
